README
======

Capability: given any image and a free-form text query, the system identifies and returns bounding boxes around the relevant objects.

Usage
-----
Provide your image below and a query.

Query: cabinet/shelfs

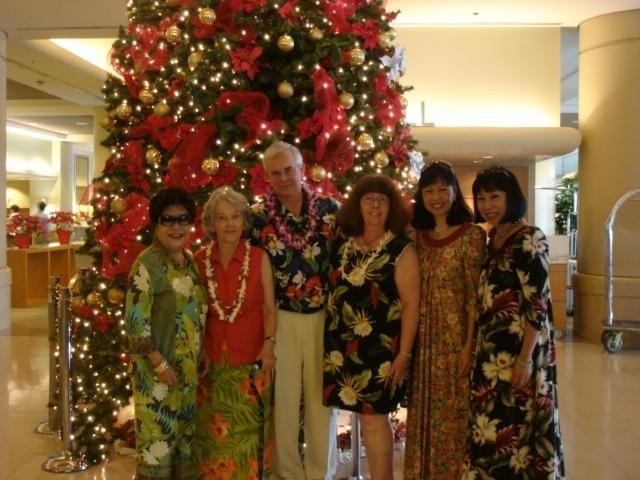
[7,241,85,308]
[549,260,577,340]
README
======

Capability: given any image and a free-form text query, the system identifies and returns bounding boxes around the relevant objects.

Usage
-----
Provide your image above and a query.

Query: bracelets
[516,355,533,366]
[153,356,169,375]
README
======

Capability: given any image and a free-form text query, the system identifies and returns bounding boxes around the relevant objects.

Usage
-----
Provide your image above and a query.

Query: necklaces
[495,218,526,238]
[434,225,448,232]
[205,239,250,324]
[361,231,386,245]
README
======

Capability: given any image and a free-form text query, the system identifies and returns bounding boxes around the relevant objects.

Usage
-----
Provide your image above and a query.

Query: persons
[6,206,26,236]
[468,167,565,480]
[405,160,487,479]
[32,202,48,230]
[248,141,344,480]
[125,186,209,480]
[322,173,420,480]
[195,186,277,480]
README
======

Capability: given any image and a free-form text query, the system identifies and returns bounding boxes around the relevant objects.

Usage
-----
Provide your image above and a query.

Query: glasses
[421,159,453,174]
[157,213,192,226]
[475,165,506,179]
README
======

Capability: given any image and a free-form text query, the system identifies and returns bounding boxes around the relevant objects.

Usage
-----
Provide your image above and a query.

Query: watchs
[264,336,275,343]
[400,351,413,357]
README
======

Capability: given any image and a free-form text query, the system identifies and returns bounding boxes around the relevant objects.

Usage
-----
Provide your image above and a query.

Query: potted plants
[7,213,46,237]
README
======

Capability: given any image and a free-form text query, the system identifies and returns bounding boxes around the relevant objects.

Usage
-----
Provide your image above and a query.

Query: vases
[13,234,32,249]
[52,230,73,245]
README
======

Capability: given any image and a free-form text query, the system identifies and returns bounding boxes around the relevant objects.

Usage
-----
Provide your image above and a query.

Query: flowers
[46,211,81,230]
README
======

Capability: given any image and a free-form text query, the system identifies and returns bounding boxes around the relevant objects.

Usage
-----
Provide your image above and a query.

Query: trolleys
[599,188,640,353]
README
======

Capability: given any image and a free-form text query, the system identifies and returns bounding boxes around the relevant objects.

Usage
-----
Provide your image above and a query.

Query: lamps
[6,119,67,142]
